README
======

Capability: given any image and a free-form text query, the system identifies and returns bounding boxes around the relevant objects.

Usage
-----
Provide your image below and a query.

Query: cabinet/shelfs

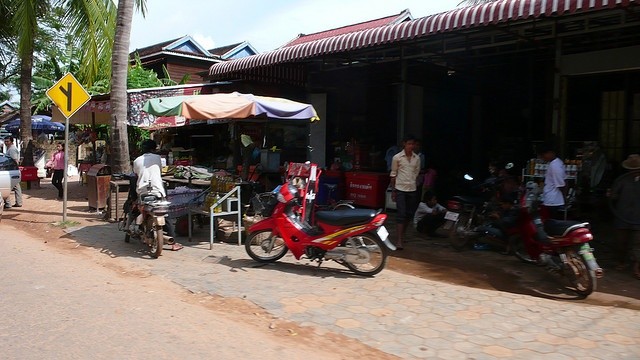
[188,185,242,251]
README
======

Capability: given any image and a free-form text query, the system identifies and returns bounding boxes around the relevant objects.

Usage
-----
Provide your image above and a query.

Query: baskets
[243,214,271,245]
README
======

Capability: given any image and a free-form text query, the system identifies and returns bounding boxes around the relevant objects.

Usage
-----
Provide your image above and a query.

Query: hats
[623,154,640,169]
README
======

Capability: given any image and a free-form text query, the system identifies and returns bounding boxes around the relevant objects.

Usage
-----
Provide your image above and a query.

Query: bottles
[563,157,584,176]
[212,173,236,193]
[204,191,214,212]
[524,158,551,176]
[213,191,223,213]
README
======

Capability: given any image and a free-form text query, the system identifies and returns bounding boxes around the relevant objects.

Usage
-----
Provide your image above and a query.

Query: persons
[539,143,566,236]
[412,191,448,235]
[384,144,400,185]
[50,142,65,198]
[388,134,421,249]
[457,195,517,247]
[3,135,23,208]
[421,160,438,201]
[414,140,425,201]
[606,154,640,279]
[131,139,185,251]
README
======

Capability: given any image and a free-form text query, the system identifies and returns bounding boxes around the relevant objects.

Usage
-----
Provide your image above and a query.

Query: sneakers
[12,204,21,207]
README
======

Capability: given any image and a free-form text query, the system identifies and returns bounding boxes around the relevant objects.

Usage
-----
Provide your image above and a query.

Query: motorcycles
[505,178,605,295]
[444,162,514,251]
[245,174,397,277]
[111,169,177,257]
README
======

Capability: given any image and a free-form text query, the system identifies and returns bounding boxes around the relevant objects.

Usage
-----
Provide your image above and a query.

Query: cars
[0,152,22,222]
